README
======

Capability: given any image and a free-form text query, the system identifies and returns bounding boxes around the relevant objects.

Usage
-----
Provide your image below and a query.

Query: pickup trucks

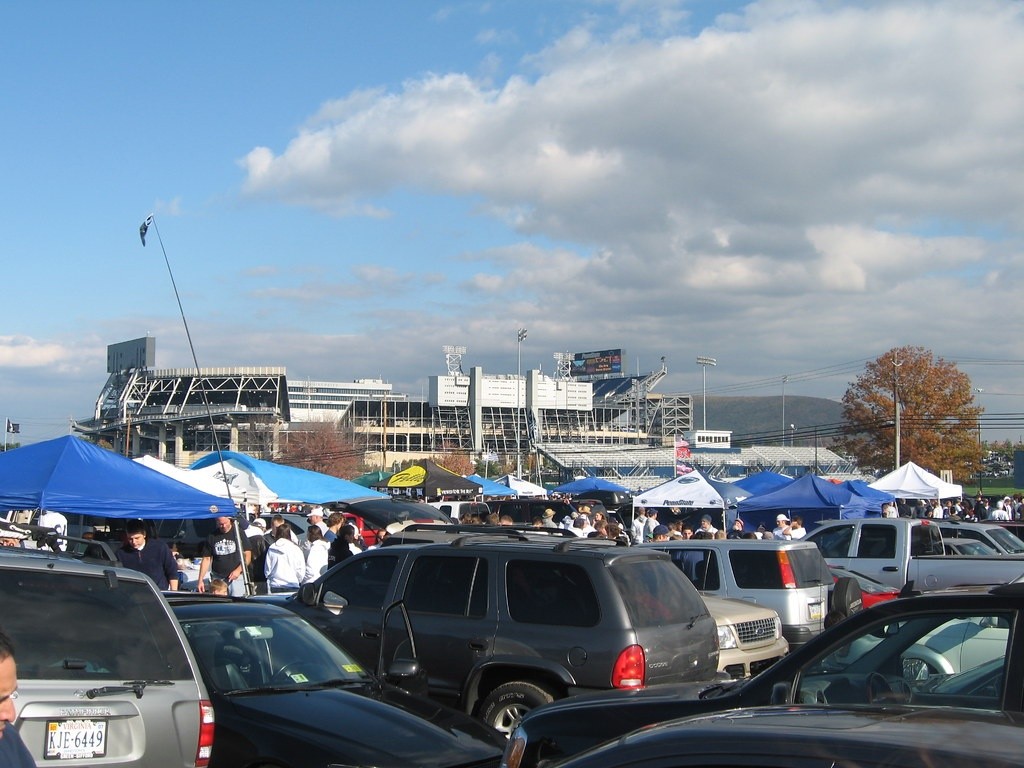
[425,502,492,525]
[799,517,1024,591]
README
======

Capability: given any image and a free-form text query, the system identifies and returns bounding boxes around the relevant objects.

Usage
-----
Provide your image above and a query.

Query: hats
[307,509,323,516]
[647,508,658,515]
[578,506,592,514]
[653,524,674,537]
[777,514,791,522]
[253,518,266,528]
[701,514,712,523]
[541,509,556,518]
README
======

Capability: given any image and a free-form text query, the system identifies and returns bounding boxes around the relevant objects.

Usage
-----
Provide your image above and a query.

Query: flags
[139,213,154,248]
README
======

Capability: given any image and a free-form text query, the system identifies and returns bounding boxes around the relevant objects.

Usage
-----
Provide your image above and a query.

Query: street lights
[517,328,528,479]
[697,356,797,447]
[974,388,982,495]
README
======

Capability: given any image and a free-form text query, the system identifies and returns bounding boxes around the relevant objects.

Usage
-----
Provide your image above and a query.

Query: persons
[114,520,178,590]
[460,505,807,549]
[237,503,387,593]
[0,632,37,768]
[209,578,228,596]
[37,510,67,552]
[197,516,251,596]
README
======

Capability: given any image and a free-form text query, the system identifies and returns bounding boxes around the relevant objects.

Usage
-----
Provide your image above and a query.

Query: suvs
[0,534,214,768]
[932,517,1024,555]
[630,540,862,653]
[252,511,334,543]
[381,524,790,682]
[245,534,730,739]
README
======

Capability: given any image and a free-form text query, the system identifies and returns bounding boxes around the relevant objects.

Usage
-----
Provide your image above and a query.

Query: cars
[63,512,219,554]
[343,513,434,551]
[834,574,1023,698]
[160,599,510,768]
[531,704,1023,768]
[826,564,901,608]
[941,538,1000,556]
[487,499,609,529]
[502,582,1024,768]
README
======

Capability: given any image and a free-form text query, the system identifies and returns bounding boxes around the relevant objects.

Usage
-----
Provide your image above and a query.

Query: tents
[737,472,893,535]
[189,451,394,510]
[349,470,395,491]
[369,458,483,502]
[1,434,247,524]
[868,460,963,502]
[635,467,752,518]
[463,472,635,498]
[719,470,895,510]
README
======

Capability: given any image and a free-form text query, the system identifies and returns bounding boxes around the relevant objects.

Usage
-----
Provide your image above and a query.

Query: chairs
[693,560,706,590]
[191,634,249,689]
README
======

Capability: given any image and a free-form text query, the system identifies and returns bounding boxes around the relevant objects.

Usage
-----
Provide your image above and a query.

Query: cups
[194,558,202,564]
